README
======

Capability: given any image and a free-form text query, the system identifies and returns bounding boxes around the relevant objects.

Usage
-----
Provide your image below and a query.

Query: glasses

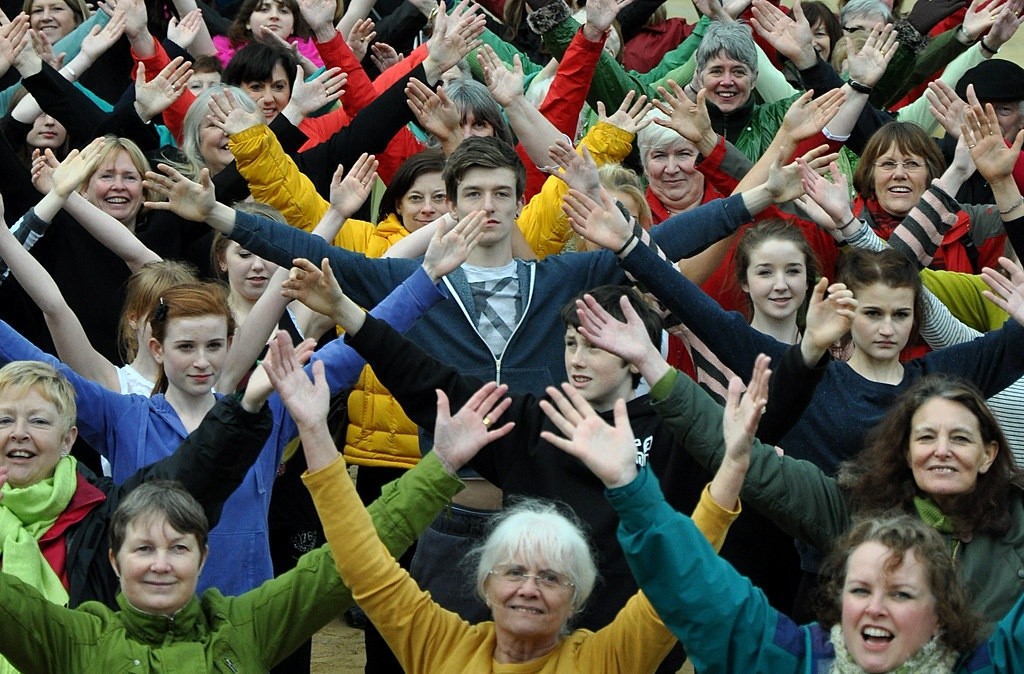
[874,159,927,175]
[843,25,882,36]
[488,565,575,594]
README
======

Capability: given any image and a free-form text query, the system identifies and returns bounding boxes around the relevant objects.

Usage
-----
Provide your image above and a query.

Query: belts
[426,507,494,539]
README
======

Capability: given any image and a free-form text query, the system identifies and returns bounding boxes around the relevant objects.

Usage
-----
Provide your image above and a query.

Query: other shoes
[347,602,367,630]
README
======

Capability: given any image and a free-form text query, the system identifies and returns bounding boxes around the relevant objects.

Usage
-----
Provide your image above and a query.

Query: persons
[0,0,1024,674]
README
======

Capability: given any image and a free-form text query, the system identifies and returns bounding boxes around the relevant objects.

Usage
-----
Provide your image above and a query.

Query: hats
[955,59,1024,106]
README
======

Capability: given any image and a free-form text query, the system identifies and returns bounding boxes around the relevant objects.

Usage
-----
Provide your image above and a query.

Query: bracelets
[838,217,855,230]
[1000,197,1024,214]
[981,39,999,54]
[429,7,439,21]
[64,66,77,81]
[847,79,871,94]
[961,26,975,39]
[614,235,636,255]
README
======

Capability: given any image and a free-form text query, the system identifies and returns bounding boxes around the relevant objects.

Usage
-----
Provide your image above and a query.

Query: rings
[172,85,176,90]
[383,59,385,61]
[992,16,995,19]
[969,145,975,148]
[668,110,672,115]
[326,90,329,95]
[880,50,885,54]
[753,404,766,414]
[228,111,231,113]
[1015,12,1019,15]
[482,419,492,426]
[361,38,364,41]
[465,40,468,43]
[361,182,366,185]
